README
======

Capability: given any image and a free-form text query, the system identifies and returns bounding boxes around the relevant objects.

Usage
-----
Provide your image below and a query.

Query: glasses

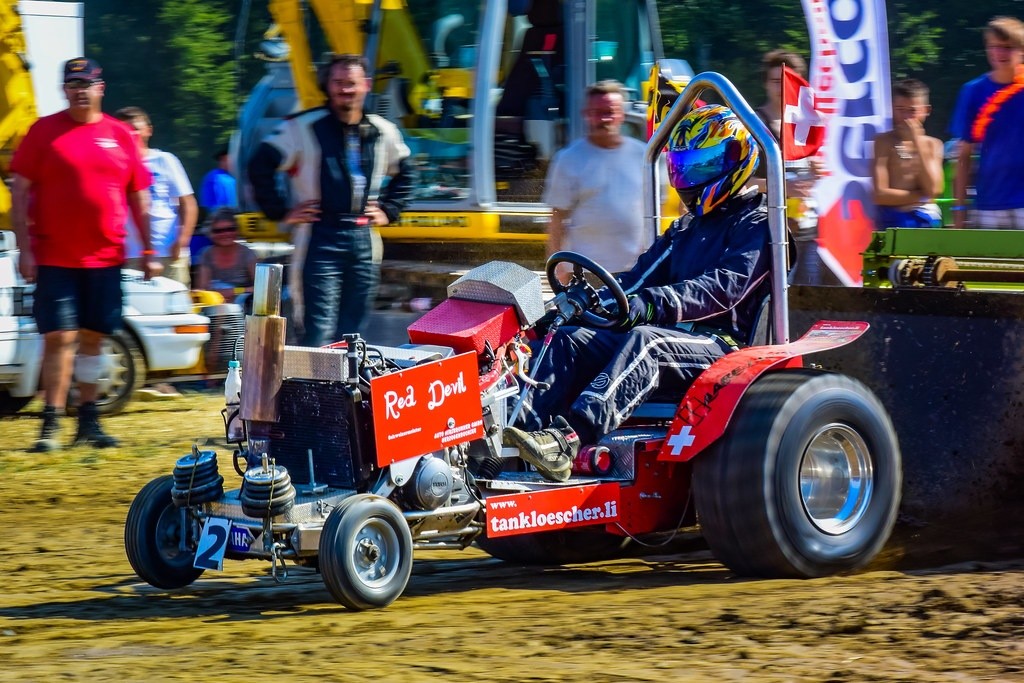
[64,79,103,89]
[211,226,236,233]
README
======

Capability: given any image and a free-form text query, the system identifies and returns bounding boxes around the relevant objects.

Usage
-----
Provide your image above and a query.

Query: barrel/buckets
[199,304,247,374]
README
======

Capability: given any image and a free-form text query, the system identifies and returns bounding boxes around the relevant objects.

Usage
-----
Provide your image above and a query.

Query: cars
[0,229,212,417]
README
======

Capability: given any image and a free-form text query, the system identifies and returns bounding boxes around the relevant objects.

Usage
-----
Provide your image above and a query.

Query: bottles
[224,360,244,440]
[569,443,617,475]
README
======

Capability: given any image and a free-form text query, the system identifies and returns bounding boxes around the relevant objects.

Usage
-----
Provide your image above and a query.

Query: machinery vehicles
[232,0,691,316]
[121,69,905,617]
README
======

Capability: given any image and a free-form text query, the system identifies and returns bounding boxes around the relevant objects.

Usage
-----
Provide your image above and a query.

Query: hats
[65,58,103,81]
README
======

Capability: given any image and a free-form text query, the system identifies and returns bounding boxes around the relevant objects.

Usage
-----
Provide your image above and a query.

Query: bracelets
[138,248,157,256]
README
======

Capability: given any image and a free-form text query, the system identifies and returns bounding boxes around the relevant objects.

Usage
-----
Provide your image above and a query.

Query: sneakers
[35,428,62,451]
[74,423,115,447]
[502,415,581,481]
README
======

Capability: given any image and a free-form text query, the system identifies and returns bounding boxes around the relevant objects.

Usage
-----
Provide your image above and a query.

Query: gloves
[611,293,658,332]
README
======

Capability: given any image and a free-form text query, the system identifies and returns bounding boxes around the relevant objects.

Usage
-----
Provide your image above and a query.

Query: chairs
[629,293,774,419]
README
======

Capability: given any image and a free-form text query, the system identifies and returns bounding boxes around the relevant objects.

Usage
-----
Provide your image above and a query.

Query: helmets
[666,104,761,218]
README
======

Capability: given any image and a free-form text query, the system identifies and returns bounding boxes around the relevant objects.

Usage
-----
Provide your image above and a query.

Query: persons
[540,79,668,282]
[10,56,164,452]
[873,78,947,231]
[950,205,967,210]
[951,15,1023,230]
[192,138,257,303]
[112,106,198,292]
[247,54,414,345]
[510,104,786,482]
[754,49,805,178]
